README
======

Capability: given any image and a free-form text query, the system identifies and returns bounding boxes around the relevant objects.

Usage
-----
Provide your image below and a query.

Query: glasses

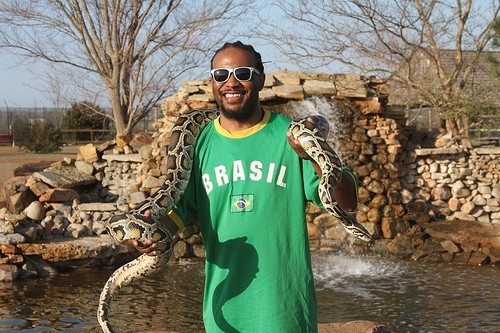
[210,67,260,83]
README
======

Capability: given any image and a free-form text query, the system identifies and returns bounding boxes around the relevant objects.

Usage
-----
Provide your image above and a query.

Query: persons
[125,41,360,333]
